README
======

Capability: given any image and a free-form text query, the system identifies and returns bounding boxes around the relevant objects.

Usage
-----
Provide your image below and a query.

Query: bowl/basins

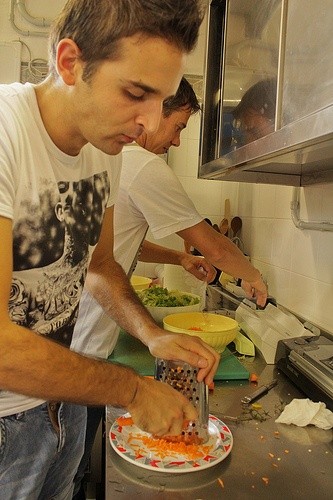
[130,276,200,323]
[162,312,239,353]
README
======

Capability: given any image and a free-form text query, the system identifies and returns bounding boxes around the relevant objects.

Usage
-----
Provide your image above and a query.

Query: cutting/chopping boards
[106,329,250,379]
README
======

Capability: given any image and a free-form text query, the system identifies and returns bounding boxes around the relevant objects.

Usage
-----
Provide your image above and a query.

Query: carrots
[115,417,214,463]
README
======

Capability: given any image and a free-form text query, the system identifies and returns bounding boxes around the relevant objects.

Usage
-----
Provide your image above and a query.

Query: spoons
[204,216,242,248]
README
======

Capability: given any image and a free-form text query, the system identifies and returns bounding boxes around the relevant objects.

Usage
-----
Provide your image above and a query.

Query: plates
[109,412,233,473]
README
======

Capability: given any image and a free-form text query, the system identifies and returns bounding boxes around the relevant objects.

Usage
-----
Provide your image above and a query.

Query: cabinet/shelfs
[197,0,333,187]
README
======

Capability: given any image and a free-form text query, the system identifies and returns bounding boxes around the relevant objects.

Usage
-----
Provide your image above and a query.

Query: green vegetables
[134,286,200,307]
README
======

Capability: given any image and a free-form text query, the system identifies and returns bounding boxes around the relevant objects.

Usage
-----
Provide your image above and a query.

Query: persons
[233,78,276,143]
[0,0,221,500]
[69,76,268,500]
